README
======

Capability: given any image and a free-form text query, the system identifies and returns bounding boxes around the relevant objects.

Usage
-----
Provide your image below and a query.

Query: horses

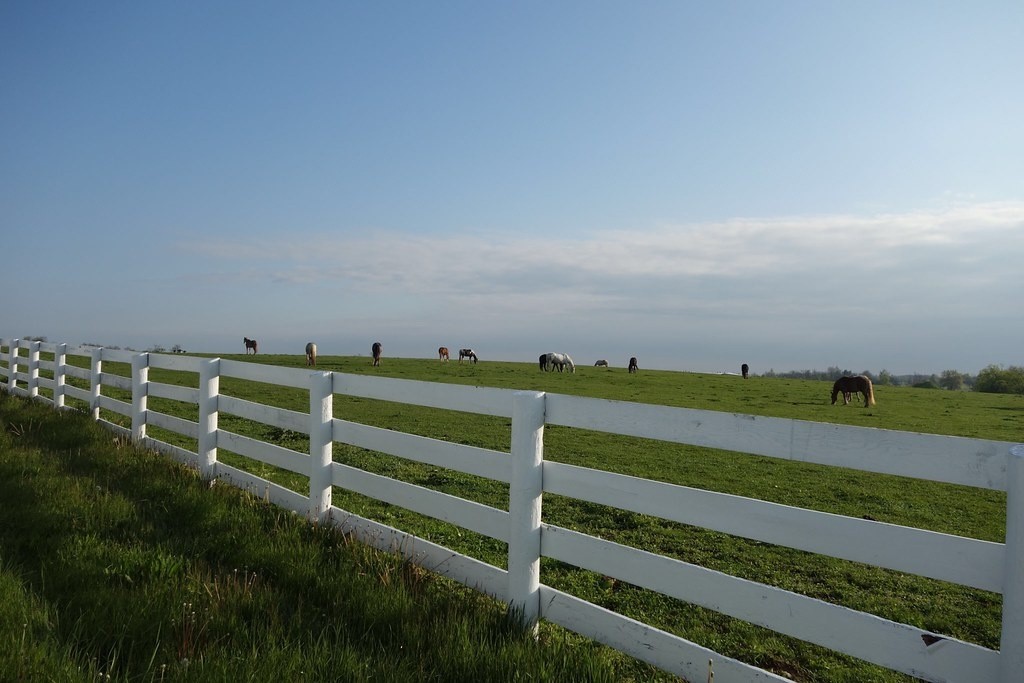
[438,346,450,362]
[594,359,608,368]
[830,373,876,409]
[741,363,749,379]
[458,347,479,363]
[371,341,382,366]
[539,351,575,374]
[305,342,317,366]
[243,336,258,354]
[627,356,639,374]
